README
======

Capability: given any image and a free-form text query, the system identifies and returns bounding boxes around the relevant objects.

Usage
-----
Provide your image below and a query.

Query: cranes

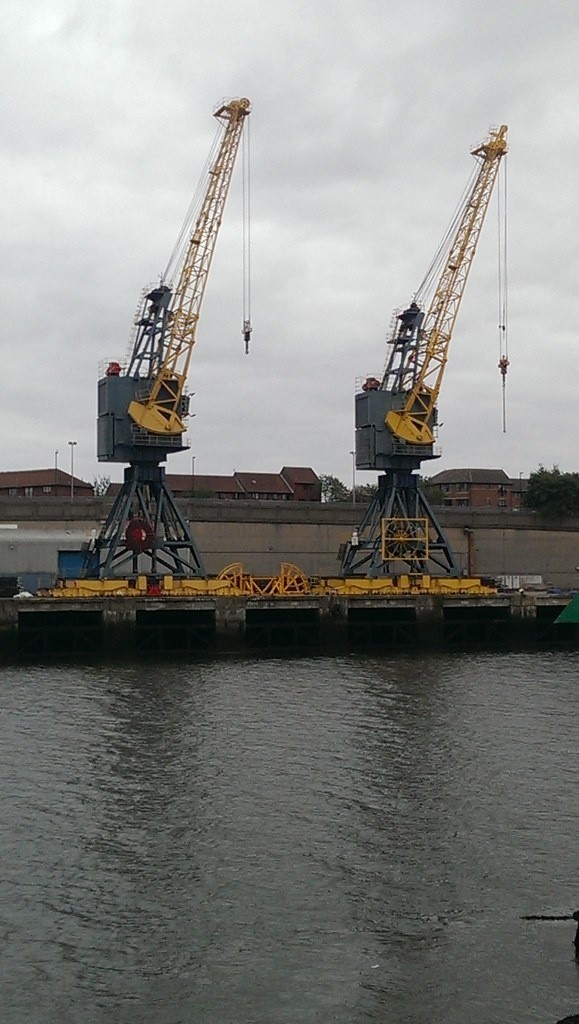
[276,125,510,595]
[41,97,256,596]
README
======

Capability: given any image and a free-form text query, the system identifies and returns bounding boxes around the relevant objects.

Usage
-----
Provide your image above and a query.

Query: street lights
[68,441,76,499]
[192,456,196,496]
[349,450,356,509]
[54,450,58,497]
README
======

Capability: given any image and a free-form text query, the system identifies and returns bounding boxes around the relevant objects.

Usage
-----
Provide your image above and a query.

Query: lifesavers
[386,521,418,556]
[124,517,155,550]
